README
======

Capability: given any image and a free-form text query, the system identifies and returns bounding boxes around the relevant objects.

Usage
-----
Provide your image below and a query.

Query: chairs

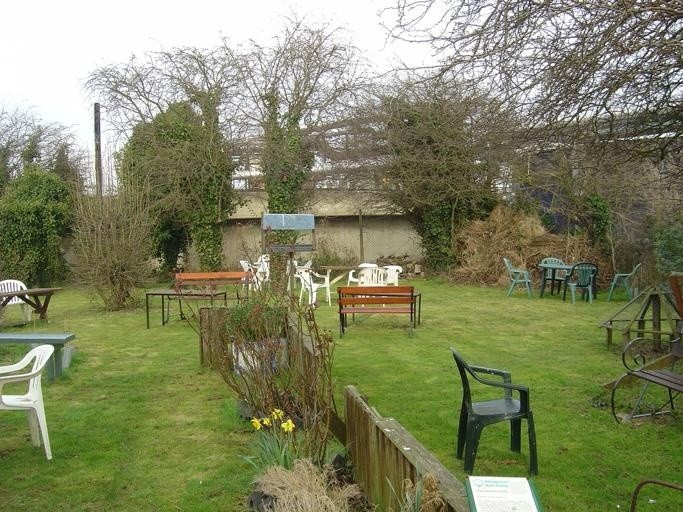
[449,346,539,476]
[239,260,263,291]
[258,254,271,283]
[563,263,598,305]
[378,266,401,286]
[0,279,32,322]
[357,267,388,308]
[296,266,329,306]
[0,343,54,461]
[503,258,533,298]
[541,258,565,295]
[168,271,254,320]
[348,263,378,287]
[611,319,683,424]
[607,262,642,304]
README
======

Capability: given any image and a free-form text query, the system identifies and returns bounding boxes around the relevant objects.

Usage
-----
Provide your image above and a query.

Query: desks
[538,264,596,299]
[0,286,61,324]
[146,288,227,330]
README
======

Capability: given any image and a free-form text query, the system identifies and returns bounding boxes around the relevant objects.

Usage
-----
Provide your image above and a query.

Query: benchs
[0,331,75,384]
